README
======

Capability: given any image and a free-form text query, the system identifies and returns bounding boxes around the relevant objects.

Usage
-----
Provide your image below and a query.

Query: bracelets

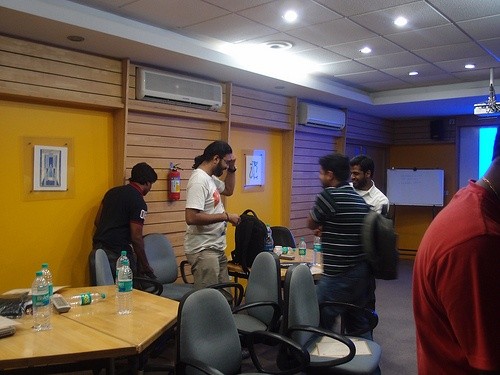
[226,212,229,222]
[228,166,236,173]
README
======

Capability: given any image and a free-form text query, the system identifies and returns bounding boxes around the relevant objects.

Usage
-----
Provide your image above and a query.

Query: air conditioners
[136,66,223,112]
[298,101,346,131]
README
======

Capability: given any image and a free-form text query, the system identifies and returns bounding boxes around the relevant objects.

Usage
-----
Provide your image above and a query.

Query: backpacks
[360,201,398,280]
[230,210,267,273]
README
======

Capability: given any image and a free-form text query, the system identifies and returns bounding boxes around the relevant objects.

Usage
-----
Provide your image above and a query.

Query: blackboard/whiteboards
[386,167,443,207]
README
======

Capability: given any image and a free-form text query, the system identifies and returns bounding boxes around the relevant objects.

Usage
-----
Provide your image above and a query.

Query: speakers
[430,120,444,139]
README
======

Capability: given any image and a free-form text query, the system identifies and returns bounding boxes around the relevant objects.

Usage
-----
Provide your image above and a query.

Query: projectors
[474,102,500,117]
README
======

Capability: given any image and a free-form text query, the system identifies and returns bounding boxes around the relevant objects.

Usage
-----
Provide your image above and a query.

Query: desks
[0,284,179,375]
[227,245,323,306]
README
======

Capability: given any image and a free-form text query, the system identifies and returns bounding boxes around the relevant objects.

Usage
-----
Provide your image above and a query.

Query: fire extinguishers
[167,162,183,201]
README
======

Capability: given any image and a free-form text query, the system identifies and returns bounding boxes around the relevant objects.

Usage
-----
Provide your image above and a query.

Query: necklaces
[483,176,500,199]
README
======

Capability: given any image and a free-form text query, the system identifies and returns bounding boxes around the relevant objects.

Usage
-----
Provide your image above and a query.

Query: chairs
[88,226,382,375]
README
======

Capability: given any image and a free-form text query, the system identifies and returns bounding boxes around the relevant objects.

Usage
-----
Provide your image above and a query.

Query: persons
[89,162,158,375]
[350,156,390,341]
[412,121,500,375]
[184,139,251,359]
[305,155,381,375]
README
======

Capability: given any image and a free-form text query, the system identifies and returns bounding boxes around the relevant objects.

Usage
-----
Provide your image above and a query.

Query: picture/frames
[245,155,262,186]
[34,145,68,192]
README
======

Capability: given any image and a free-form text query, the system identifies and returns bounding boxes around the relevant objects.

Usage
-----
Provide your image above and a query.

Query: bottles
[298,238,307,264]
[40,263,54,314]
[312,233,322,267]
[31,272,52,331]
[266,224,274,247]
[114,251,130,290]
[115,260,133,315]
[70,293,106,306]
[273,246,296,255]
